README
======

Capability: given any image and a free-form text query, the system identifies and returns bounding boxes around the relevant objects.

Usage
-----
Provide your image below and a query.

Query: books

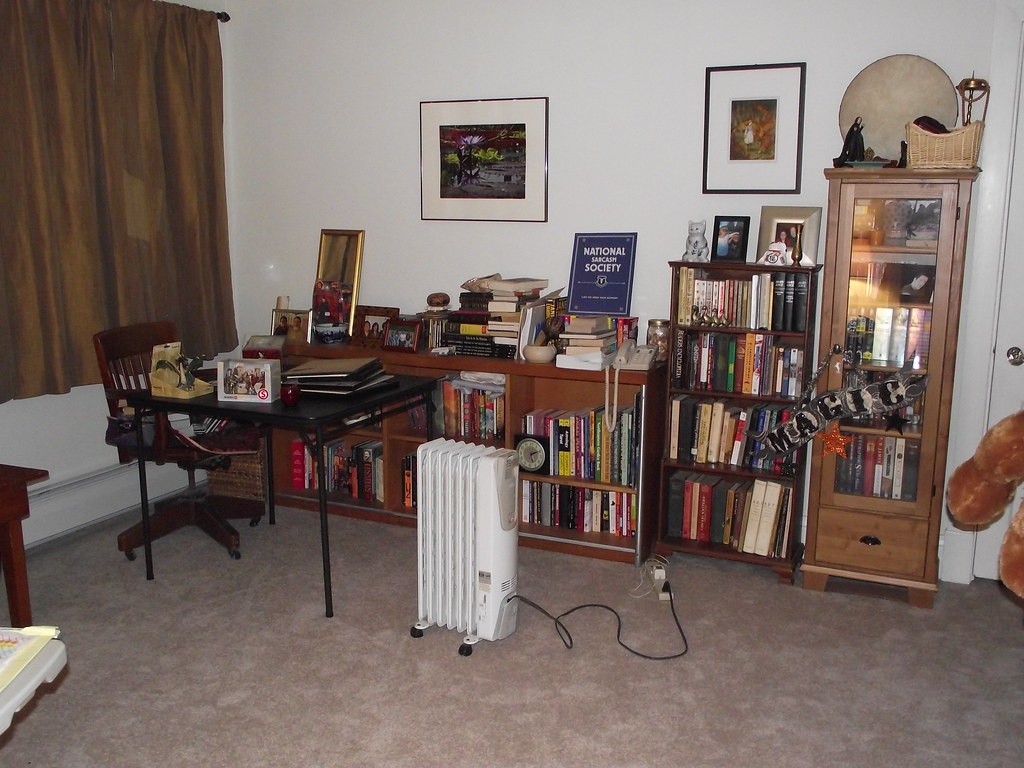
[677,267,808,332]
[406,380,505,440]
[842,370,922,424]
[522,479,637,537]
[847,305,932,370]
[428,278,618,371]
[521,392,641,488]
[291,434,385,504]
[666,470,793,559]
[402,452,417,507]
[673,329,804,398]
[669,394,793,470]
[836,432,920,500]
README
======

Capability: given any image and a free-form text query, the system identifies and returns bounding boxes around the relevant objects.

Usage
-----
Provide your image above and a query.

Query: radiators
[411,437,521,657]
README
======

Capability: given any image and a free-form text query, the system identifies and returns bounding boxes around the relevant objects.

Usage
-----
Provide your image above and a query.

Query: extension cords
[645,561,674,600]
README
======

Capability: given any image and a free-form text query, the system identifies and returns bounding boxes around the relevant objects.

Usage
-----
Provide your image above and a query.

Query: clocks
[514,433,551,476]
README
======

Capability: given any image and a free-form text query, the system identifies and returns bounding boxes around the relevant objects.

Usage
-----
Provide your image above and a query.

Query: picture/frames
[353,304,399,345]
[383,319,423,354]
[756,206,822,267]
[420,96,548,222]
[711,216,750,264]
[271,309,313,344]
[702,62,807,194]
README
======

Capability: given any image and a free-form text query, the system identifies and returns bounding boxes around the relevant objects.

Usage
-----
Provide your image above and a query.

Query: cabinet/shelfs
[274,341,665,566]
[656,261,823,585]
[800,168,982,609]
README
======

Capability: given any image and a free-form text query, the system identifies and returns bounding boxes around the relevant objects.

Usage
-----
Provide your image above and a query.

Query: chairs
[93,319,267,561]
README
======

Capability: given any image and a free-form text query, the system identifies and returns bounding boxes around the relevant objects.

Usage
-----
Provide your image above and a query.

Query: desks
[0,464,49,629]
[128,375,443,617]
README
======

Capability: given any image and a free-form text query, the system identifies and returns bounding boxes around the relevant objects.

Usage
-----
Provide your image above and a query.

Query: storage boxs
[217,359,281,403]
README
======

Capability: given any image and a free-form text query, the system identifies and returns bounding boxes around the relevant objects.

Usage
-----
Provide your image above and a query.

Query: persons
[717,221,739,257]
[364,320,386,337]
[388,331,411,347]
[902,272,929,295]
[776,226,797,247]
[273,315,308,341]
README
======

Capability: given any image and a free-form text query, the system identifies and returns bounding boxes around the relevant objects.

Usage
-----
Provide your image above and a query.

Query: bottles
[646,318,670,363]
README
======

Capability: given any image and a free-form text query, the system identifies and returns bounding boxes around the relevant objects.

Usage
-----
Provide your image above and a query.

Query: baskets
[905,121,985,170]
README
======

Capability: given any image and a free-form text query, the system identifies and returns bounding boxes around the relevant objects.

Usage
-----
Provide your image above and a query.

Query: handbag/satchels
[105,413,157,449]
[833,116,865,168]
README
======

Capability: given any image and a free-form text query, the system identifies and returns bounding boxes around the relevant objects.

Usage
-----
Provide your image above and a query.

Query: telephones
[612,339,660,371]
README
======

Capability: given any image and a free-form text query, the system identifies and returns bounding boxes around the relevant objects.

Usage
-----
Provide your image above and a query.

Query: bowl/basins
[523,345,557,364]
